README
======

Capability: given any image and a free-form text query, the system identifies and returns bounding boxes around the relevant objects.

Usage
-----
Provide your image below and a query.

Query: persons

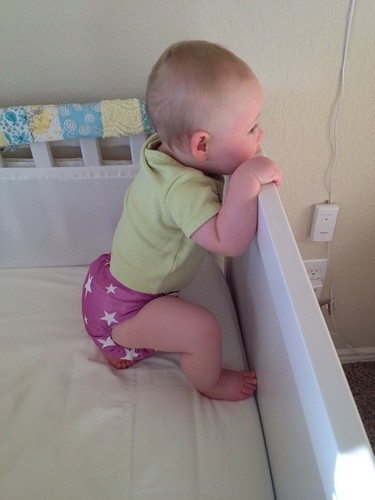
[81,40,282,402]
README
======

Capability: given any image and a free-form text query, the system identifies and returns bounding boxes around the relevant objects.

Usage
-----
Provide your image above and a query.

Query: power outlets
[305,259,327,298]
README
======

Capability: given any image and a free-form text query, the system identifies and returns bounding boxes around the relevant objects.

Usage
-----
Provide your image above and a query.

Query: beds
[0,257,276,500]
